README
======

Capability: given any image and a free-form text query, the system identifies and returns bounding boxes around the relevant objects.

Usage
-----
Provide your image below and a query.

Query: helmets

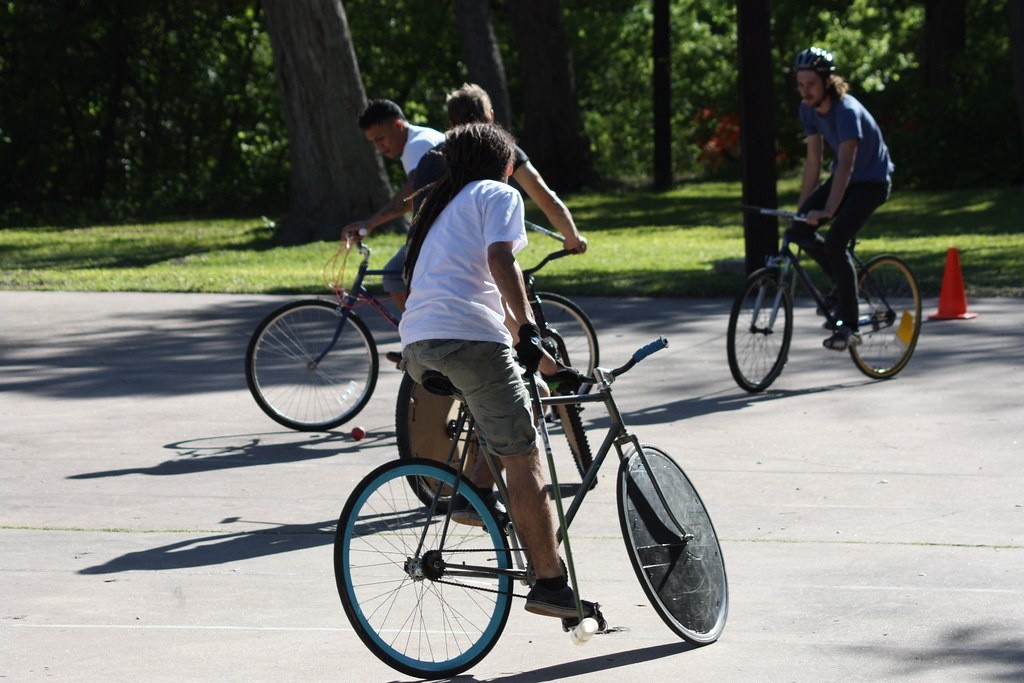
[795,47,836,74]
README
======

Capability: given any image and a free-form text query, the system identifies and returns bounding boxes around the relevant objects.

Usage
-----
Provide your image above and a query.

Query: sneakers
[525,584,590,618]
[823,327,863,350]
[451,497,505,526]
[824,312,847,329]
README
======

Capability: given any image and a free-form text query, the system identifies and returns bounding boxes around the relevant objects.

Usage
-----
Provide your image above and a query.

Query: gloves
[540,360,582,395]
[514,324,543,378]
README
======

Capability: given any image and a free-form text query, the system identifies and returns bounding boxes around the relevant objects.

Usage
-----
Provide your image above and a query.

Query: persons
[409,79,583,294]
[340,97,445,363]
[783,46,895,351]
[398,123,590,620]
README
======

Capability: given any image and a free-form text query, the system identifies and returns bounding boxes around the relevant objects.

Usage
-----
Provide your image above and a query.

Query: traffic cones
[924,245,982,319]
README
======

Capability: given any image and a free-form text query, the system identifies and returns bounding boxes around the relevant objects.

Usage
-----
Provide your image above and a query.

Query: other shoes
[386,351,403,362]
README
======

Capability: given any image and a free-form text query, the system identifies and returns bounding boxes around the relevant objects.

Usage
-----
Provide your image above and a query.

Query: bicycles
[334,331,728,681]
[394,247,597,514]
[726,201,923,392]
[244,229,600,433]
